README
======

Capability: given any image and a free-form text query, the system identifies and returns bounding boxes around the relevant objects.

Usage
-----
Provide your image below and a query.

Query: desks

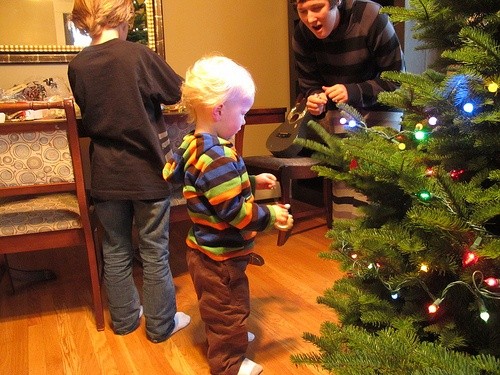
[1,106,286,296]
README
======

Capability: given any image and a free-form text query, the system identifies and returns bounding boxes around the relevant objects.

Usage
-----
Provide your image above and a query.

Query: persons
[293,1,411,232]
[162,50,293,375]
[68,1,191,344]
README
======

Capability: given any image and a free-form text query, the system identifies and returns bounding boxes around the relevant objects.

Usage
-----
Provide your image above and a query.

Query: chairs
[1,99,105,332]
[234,93,333,246]
[131,112,195,273]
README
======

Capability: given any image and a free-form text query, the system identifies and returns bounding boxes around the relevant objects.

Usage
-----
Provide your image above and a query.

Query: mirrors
[0,0,166,65]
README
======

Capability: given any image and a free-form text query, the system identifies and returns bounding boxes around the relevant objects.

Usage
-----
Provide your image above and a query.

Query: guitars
[266,98,312,158]
